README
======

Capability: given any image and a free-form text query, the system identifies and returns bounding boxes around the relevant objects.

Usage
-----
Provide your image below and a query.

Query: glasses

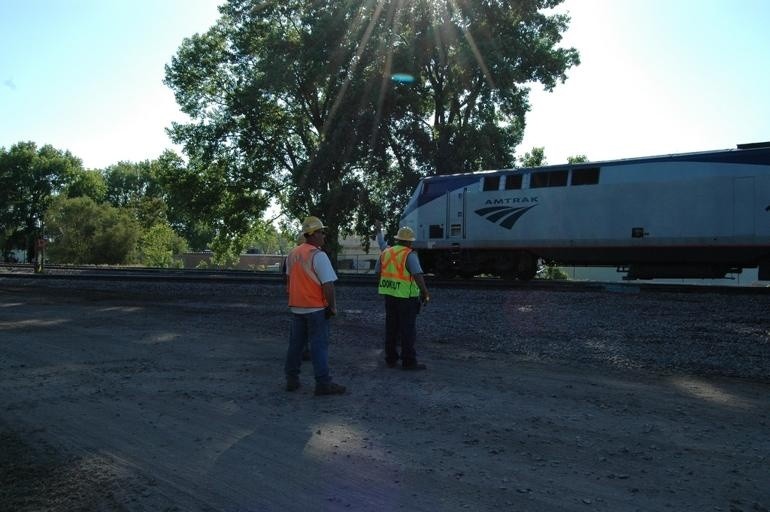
[317,231,325,235]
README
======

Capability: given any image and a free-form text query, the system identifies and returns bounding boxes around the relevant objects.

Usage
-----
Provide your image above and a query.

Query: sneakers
[386,361,426,369]
[302,352,312,361]
[315,384,345,395]
[287,383,300,391]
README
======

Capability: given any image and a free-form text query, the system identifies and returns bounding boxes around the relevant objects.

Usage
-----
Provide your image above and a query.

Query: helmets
[302,216,328,234]
[394,227,416,241]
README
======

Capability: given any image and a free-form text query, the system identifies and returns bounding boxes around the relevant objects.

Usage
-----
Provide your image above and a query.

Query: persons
[282,231,312,370]
[374,226,433,369]
[284,215,349,397]
[375,220,390,250]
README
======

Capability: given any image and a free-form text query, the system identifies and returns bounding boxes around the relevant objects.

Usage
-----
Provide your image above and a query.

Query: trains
[396,141,770,285]
